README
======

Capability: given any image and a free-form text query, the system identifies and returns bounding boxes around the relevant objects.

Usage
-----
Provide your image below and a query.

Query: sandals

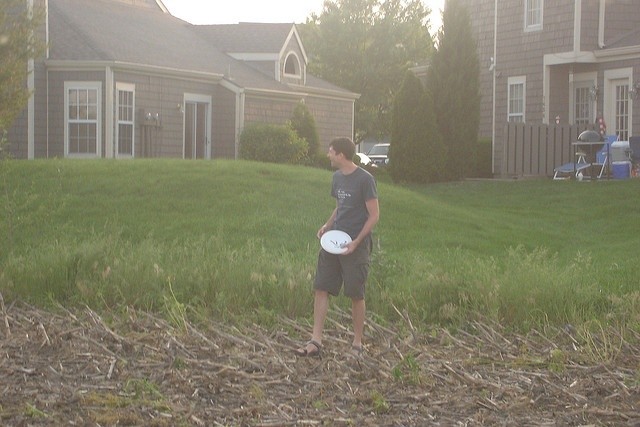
[346,345,362,361]
[295,340,322,356]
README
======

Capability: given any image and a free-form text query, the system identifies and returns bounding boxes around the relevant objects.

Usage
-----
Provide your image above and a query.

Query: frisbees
[320,230,353,256]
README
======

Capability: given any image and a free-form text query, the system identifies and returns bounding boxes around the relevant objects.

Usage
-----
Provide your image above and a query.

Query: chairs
[553,134,621,180]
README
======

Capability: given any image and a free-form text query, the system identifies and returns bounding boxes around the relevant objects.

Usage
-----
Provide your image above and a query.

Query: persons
[295,137,380,361]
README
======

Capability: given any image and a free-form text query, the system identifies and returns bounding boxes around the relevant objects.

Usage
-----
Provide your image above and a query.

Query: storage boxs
[612,161,631,178]
[611,140,628,162]
